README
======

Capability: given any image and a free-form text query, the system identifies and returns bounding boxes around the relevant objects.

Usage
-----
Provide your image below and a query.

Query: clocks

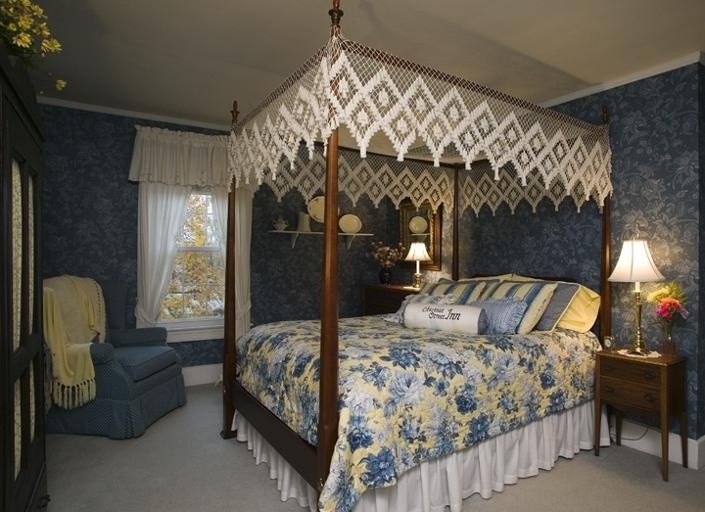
[602,335,616,352]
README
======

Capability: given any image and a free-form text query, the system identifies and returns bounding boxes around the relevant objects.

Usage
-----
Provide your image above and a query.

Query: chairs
[43,273,186,441]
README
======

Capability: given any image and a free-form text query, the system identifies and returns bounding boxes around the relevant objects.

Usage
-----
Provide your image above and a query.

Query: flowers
[0,0,69,94]
[645,276,692,329]
[357,239,407,269]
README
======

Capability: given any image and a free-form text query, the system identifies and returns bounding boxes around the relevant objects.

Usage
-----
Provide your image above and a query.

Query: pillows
[402,273,601,336]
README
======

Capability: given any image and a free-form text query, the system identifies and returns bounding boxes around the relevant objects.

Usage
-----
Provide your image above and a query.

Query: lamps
[606,236,668,357]
[403,241,433,287]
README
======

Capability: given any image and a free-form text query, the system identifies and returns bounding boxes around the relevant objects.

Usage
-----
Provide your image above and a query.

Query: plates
[308,196,341,223]
[408,215,428,233]
[339,214,362,233]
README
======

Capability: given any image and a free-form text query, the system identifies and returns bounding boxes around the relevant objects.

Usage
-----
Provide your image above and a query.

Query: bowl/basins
[408,234,429,243]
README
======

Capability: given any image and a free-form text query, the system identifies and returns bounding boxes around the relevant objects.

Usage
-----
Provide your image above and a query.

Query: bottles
[298,211,313,233]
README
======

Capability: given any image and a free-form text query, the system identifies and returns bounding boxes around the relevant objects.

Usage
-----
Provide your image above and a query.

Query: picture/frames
[396,196,442,272]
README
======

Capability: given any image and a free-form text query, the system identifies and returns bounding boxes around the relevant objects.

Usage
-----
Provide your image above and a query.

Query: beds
[218,1,613,512]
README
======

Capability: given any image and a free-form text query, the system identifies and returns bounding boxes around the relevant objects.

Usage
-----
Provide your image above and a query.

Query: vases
[379,268,392,285]
[662,320,679,358]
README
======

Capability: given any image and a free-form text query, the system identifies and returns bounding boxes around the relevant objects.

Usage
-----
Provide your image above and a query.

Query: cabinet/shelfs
[0,54,51,512]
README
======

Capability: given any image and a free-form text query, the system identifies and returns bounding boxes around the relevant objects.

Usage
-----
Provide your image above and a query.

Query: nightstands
[369,282,420,315]
[592,345,689,482]
